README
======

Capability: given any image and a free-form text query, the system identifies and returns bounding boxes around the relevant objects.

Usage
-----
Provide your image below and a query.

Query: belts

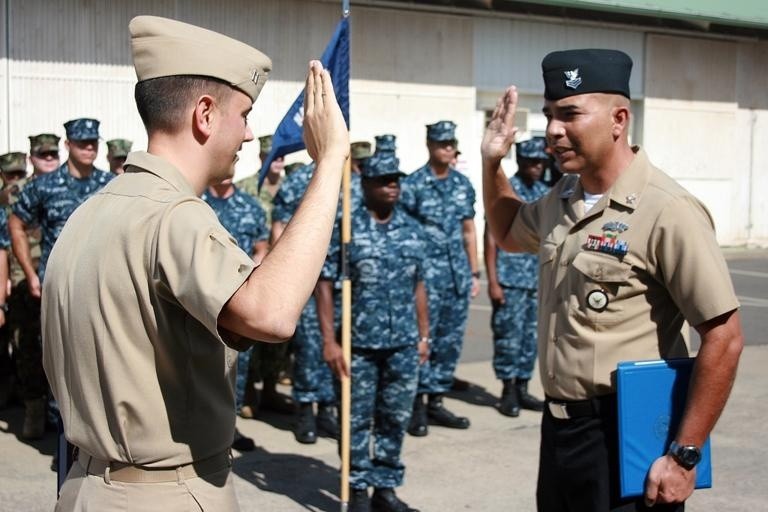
[77,448,231,484]
[542,396,618,420]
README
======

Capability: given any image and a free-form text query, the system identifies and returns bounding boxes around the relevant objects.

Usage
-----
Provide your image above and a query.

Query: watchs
[668,443,705,469]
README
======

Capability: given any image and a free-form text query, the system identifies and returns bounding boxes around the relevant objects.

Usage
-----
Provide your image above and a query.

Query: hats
[514,138,550,160]
[258,134,273,154]
[351,134,407,177]
[541,48,633,102]
[283,162,305,176]
[425,120,457,141]
[127,14,273,104]
[2,119,133,172]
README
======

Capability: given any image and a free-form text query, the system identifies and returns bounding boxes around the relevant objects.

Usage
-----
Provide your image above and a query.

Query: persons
[484,137,553,416]
[202,118,481,512]
[482,48,744,512]
[0,118,132,439]
[42,15,354,512]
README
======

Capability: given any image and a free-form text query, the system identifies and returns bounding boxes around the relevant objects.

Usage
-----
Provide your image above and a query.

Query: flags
[255,12,350,192]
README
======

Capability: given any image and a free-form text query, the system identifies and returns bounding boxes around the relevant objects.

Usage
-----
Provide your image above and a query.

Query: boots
[371,487,420,512]
[346,487,372,512]
[249,376,548,441]
[22,398,46,438]
